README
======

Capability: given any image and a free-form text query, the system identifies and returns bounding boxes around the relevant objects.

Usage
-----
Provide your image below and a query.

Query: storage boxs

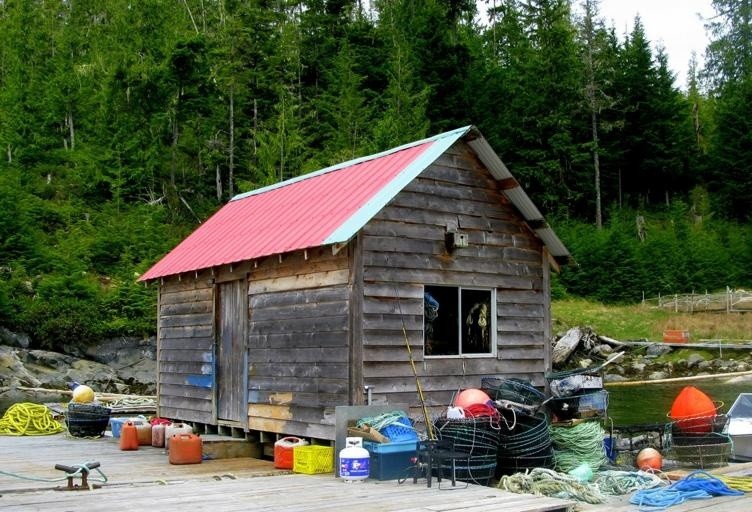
[363,440,420,481]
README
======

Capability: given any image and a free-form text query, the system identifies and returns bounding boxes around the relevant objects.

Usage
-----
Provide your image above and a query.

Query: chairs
[413,440,471,488]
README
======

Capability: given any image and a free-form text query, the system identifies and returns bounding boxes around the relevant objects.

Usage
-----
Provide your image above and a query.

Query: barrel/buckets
[169,432,203,464]
[135,421,152,446]
[121,423,138,451]
[152,423,165,448]
[274,437,308,470]
[165,423,193,450]
